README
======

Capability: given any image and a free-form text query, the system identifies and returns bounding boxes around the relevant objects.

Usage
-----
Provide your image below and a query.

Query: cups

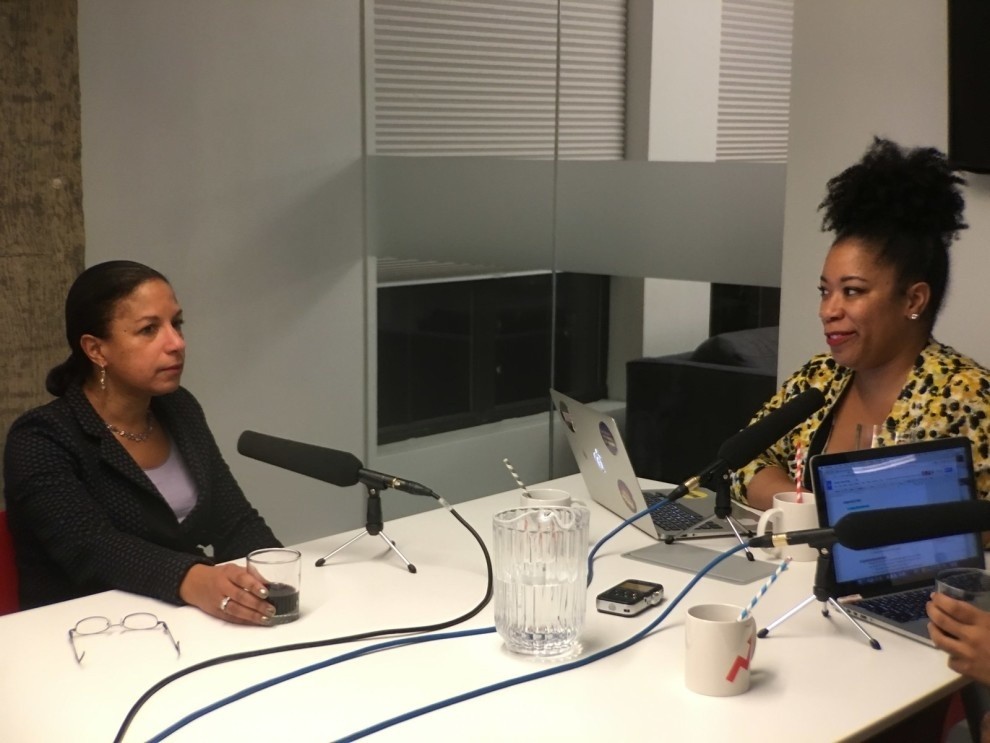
[757,492,820,562]
[933,567,990,640]
[520,489,586,509]
[684,603,758,696]
[247,548,302,624]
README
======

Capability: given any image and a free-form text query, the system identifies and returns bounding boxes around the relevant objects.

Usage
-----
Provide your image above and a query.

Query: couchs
[625,326,776,491]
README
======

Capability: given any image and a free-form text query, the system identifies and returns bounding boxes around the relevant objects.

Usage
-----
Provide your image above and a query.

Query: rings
[220,596,230,610]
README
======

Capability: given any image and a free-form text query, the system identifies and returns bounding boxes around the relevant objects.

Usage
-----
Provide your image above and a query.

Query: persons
[718,135,990,545]
[926,591,990,685]
[6,260,281,626]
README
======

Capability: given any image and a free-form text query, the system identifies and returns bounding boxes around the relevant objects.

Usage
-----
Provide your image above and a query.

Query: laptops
[808,437,986,647]
[548,389,774,542]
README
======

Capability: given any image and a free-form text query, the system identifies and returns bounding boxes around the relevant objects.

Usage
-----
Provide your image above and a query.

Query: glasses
[68,612,181,663]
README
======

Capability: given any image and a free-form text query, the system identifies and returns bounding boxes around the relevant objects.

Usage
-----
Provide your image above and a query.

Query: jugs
[491,505,591,656]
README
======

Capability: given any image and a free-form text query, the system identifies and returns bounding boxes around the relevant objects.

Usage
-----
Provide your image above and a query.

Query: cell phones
[596,579,663,615]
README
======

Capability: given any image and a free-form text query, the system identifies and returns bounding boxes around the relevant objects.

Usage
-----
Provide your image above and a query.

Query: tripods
[663,479,754,562]
[755,546,883,651]
[315,488,417,573]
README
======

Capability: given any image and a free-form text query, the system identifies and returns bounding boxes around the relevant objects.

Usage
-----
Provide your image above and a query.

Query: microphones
[749,499,990,550]
[236,431,432,496]
[671,389,827,500]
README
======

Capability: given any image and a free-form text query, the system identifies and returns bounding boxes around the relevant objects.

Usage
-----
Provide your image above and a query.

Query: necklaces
[104,409,156,444]
[849,384,902,449]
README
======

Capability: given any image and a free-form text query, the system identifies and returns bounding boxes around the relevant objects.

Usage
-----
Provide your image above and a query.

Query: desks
[1,472,990,743]
